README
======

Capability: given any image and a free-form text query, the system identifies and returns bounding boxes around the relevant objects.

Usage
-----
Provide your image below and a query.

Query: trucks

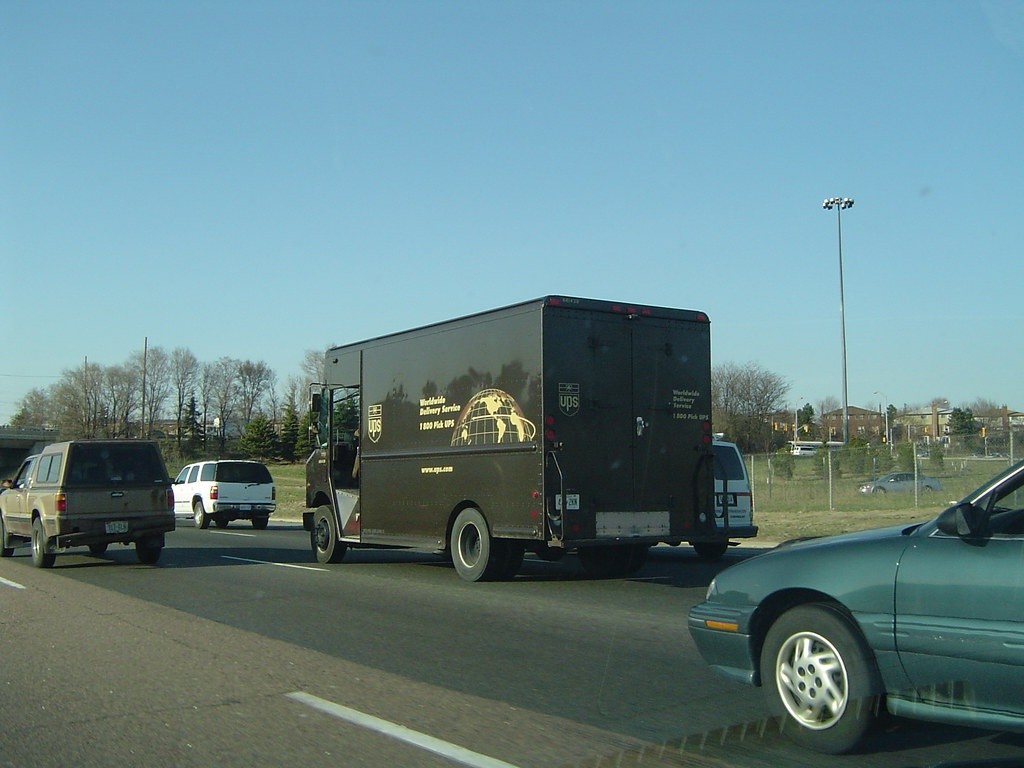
[301,295,713,582]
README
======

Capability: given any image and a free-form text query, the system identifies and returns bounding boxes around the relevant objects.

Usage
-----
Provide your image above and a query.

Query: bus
[788,440,845,456]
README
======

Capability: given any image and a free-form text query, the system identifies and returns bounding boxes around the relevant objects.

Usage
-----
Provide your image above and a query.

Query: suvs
[168,458,277,530]
[711,433,759,558]
[0,440,176,570]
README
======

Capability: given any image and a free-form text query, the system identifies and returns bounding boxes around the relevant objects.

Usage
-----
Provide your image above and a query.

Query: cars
[858,471,940,495]
[687,461,1024,755]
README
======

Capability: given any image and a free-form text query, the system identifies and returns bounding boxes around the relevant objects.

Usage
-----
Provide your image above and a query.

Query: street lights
[822,197,856,446]
[795,397,803,441]
[873,391,890,445]
